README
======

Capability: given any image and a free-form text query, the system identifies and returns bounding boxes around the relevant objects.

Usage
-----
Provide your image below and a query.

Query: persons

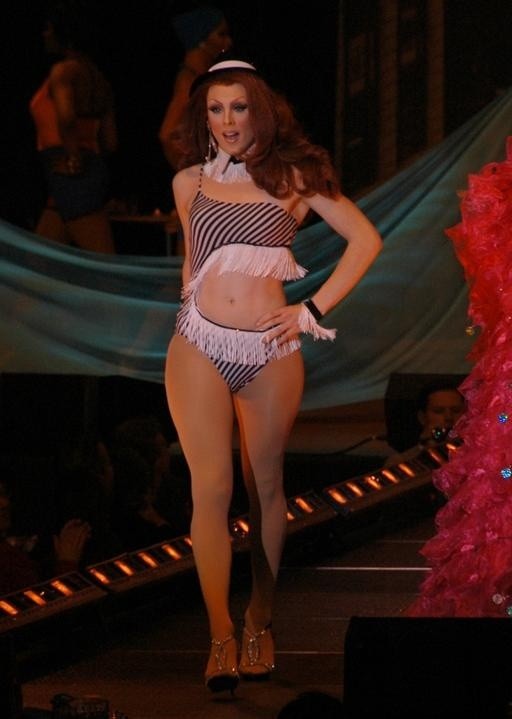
[30,9,116,262]
[161,7,230,238]
[387,390,465,467]
[164,61,382,691]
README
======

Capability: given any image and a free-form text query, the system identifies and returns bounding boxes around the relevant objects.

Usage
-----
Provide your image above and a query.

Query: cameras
[432,426,453,443]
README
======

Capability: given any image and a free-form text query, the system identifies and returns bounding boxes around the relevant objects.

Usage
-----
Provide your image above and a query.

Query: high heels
[238,607,276,683]
[204,623,241,695]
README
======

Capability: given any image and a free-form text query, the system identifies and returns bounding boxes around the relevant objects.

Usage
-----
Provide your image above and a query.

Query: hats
[189,59,258,98]
